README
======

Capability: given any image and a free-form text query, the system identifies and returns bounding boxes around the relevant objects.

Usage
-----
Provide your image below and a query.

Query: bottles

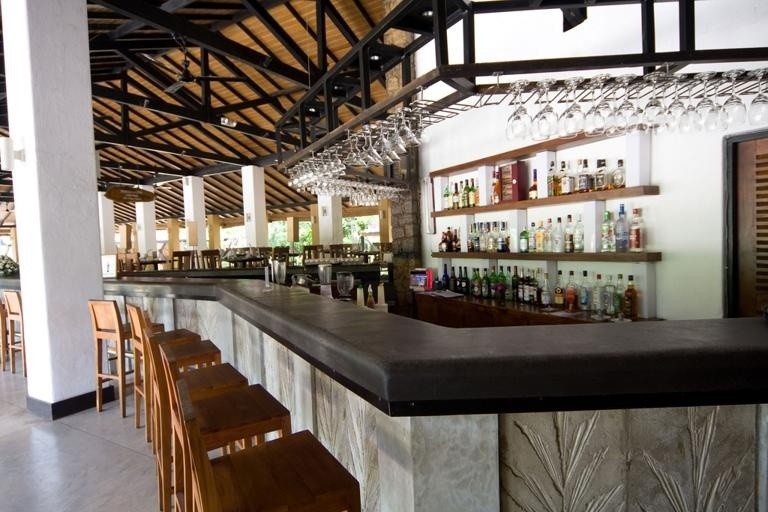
[456,227,461,252]
[518,268,524,305]
[562,162,575,194]
[505,266,512,304]
[474,221,498,254]
[591,274,603,314]
[573,214,584,254]
[490,266,498,299]
[468,179,475,207]
[579,159,594,192]
[525,269,530,302]
[442,263,448,290]
[467,225,474,251]
[551,217,563,254]
[495,172,501,204]
[600,211,616,253]
[451,229,457,251]
[614,274,623,318]
[614,204,630,253]
[535,220,546,254]
[448,181,453,209]
[530,268,542,304]
[443,184,448,211]
[564,214,573,254]
[565,271,578,312]
[593,159,611,191]
[624,275,637,320]
[538,273,552,308]
[446,227,451,251]
[458,182,464,208]
[578,271,591,310]
[512,266,517,305]
[461,267,470,294]
[573,159,584,193]
[553,270,565,309]
[529,223,536,254]
[506,221,510,251]
[609,159,626,189]
[463,180,468,208]
[482,268,489,298]
[603,275,616,315]
[544,219,554,252]
[498,220,507,251]
[456,267,462,292]
[470,268,481,296]
[528,169,538,199]
[449,266,456,291]
[438,232,448,252]
[357,283,364,307]
[556,161,568,196]
[491,170,496,204]
[498,266,505,302]
[476,178,479,206]
[547,161,559,196]
[627,209,644,253]
[452,182,458,209]
[519,227,528,253]
[366,284,375,308]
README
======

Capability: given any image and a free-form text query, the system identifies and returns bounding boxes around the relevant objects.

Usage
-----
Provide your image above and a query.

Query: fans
[135,36,250,94]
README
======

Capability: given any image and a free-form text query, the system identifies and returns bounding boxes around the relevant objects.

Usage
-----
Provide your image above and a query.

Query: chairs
[272,245,290,266]
[88,299,165,418]
[158,342,292,512]
[175,379,361,512]
[117,259,122,272]
[143,310,221,455]
[0,298,9,372]
[239,248,250,254]
[142,327,248,512]
[126,259,140,270]
[3,291,27,378]
[171,249,200,270]
[259,247,272,267]
[303,245,325,263]
[354,251,380,263]
[329,243,352,258]
[125,303,201,443]
[120,259,126,270]
[201,250,221,269]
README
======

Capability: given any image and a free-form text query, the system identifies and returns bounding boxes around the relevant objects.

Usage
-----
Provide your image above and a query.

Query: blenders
[272,255,286,284]
[336,272,354,300]
[318,264,333,299]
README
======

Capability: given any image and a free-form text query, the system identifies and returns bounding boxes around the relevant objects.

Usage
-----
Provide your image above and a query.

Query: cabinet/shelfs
[415,291,666,326]
